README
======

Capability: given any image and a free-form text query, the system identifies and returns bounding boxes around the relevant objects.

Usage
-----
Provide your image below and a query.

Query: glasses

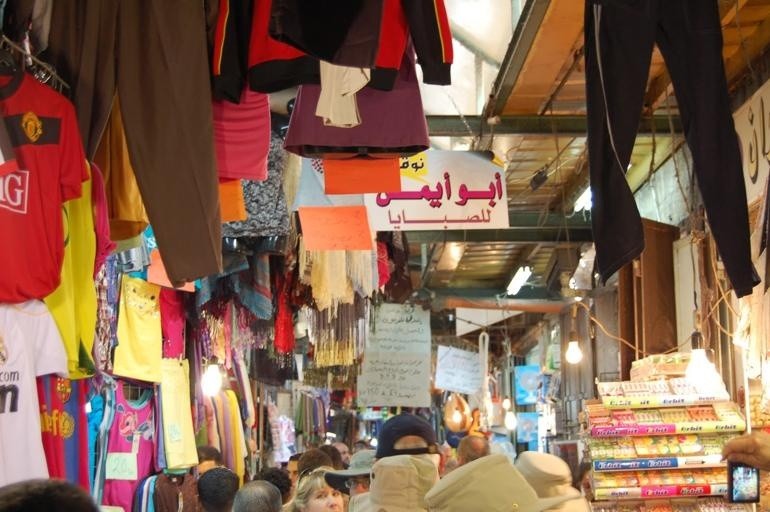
[343,477,368,489]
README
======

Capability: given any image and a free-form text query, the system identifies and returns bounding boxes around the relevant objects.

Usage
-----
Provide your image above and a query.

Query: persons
[720,428,770,472]
[191,413,595,512]
[0,479,99,512]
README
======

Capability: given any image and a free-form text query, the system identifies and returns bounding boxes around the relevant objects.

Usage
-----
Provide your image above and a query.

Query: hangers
[0,35,64,94]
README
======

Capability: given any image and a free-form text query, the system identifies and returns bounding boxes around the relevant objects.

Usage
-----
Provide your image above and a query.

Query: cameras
[727,459,760,502]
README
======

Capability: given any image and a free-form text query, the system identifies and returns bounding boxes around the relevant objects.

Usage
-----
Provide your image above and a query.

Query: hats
[350,450,593,512]
[374,412,440,460]
[324,449,376,491]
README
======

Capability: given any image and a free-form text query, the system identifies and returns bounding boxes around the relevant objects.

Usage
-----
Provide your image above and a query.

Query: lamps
[566,300,582,364]
[505,249,534,295]
[567,173,592,214]
